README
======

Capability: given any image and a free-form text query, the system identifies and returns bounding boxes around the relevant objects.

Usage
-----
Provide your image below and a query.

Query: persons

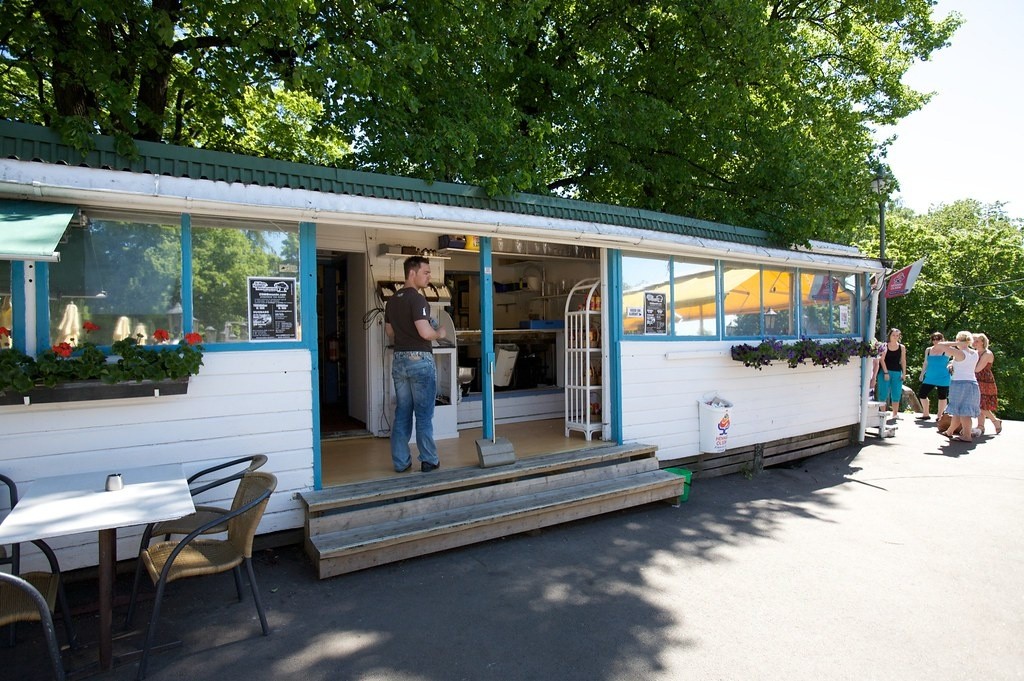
[915,331,958,421]
[869,327,906,420]
[938,330,981,443]
[385,256,447,473]
[970,333,1002,438]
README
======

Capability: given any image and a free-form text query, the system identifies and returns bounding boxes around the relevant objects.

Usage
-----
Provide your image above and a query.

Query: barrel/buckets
[664,468,692,501]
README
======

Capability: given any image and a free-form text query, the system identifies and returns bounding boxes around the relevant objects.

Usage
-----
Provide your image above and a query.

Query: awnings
[0,197,78,263]
[622,268,850,332]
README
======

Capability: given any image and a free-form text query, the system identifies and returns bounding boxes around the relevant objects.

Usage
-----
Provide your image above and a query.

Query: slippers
[951,437,972,442]
[941,432,953,438]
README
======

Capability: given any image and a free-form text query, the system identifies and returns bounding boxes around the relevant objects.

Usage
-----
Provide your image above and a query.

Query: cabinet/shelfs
[565,303,603,431]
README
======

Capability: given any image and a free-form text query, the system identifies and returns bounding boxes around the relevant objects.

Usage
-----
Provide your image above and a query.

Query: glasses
[932,338,941,341]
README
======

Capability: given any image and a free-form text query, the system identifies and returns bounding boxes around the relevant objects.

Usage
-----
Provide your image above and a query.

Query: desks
[1,479,197,681]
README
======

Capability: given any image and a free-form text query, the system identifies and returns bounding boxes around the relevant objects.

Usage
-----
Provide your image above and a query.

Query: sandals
[977,425,985,435]
[993,419,1002,433]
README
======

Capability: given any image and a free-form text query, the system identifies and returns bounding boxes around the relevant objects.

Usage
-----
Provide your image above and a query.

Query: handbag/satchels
[937,403,963,434]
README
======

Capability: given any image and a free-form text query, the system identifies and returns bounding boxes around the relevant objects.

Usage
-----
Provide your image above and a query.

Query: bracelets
[884,373,888,374]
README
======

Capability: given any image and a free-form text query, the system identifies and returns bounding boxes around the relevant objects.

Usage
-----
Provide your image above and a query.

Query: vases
[0,367,190,407]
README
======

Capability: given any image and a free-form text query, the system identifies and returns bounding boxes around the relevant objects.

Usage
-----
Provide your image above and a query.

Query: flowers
[0,322,206,395]
[731,335,888,370]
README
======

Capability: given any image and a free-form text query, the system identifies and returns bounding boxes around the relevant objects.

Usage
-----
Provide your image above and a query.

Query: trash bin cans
[493,344,519,386]
[698,397,733,454]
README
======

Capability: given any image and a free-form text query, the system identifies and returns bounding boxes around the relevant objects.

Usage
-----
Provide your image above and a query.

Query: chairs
[0,472,76,680]
[125,455,277,681]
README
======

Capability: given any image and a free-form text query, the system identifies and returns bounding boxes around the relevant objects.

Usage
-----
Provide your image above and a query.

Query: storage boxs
[379,244,419,254]
[519,318,562,330]
[377,280,452,302]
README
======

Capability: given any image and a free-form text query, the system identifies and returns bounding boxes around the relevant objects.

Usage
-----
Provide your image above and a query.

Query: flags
[885,257,926,298]
[808,274,839,302]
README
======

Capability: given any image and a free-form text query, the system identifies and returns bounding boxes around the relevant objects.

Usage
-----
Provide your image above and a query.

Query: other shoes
[421,460,440,472]
[892,415,898,420]
[917,416,930,420]
[397,463,411,472]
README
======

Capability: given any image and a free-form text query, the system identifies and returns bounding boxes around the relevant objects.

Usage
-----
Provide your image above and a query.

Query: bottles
[456,328,481,345]
[519,276,527,288]
[430,318,439,329]
